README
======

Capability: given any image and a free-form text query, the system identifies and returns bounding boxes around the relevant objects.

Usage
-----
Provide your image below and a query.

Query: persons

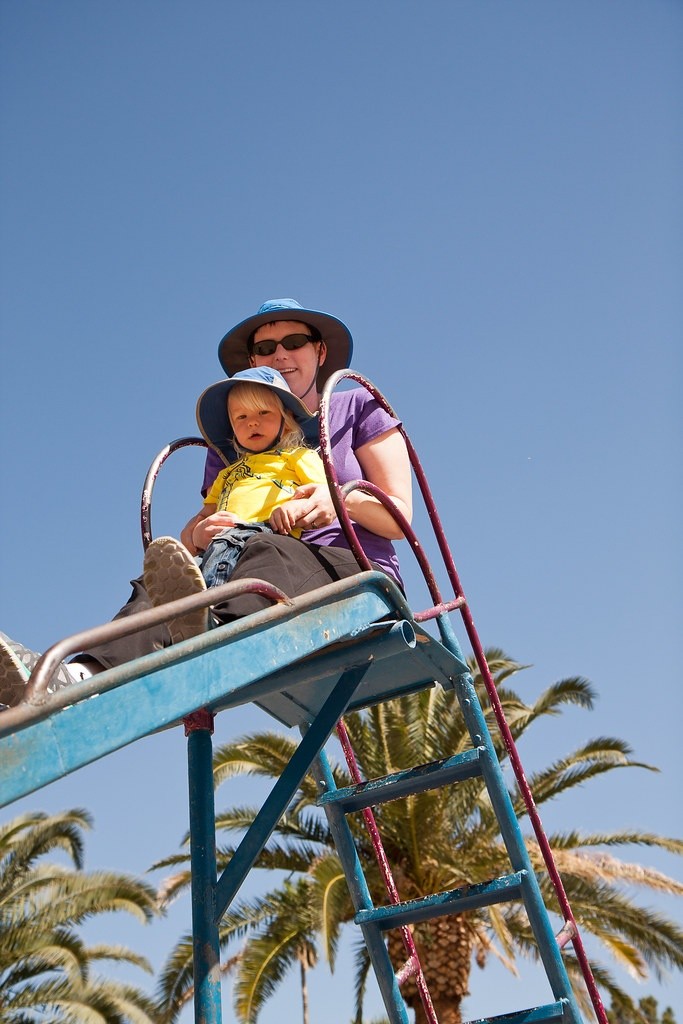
[195,367,328,590]
[0,298,414,710]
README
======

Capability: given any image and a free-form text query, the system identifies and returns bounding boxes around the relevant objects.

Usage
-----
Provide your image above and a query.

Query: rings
[311,522,317,527]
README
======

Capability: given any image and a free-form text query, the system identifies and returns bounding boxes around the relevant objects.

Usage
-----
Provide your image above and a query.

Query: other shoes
[142,536,210,646]
[0,631,95,718]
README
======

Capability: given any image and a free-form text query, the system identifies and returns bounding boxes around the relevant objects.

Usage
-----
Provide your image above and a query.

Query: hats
[196,367,321,467]
[218,298,353,394]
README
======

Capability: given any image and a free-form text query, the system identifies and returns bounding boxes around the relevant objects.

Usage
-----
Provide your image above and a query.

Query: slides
[0,584,397,812]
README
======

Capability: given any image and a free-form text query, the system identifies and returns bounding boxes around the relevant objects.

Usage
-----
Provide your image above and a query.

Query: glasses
[253,334,319,356]
